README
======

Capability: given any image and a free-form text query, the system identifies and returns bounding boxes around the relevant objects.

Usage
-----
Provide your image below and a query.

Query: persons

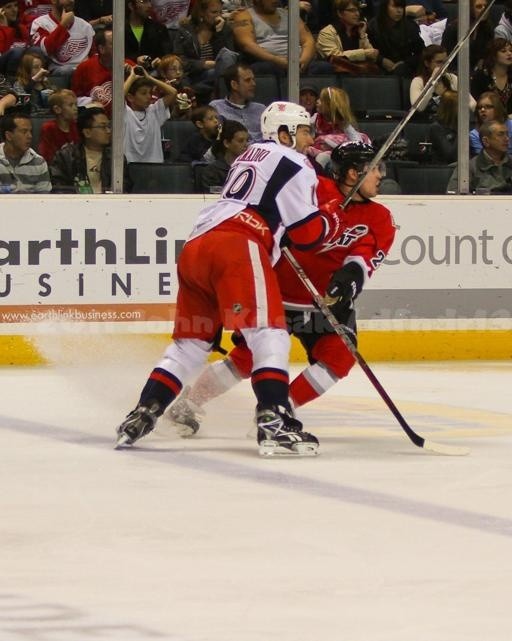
[125,0,171,66]
[440,0,498,70]
[404,0,458,71]
[0,71,19,117]
[471,37,512,114]
[14,53,59,118]
[173,0,246,105]
[299,79,320,115]
[0,112,53,194]
[123,65,177,194]
[155,54,197,121]
[0,0,49,77]
[198,120,248,194]
[446,120,512,195]
[73,30,139,119]
[114,102,348,457]
[233,0,337,101]
[164,140,396,437]
[367,0,425,76]
[409,44,477,123]
[468,92,512,151]
[208,63,266,143]
[309,86,371,172]
[49,107,113,194]
[39,88,78,165]
[494,0,512,45]
[175,105,219,163]
[299,0,340,34]
[316,0,384,77]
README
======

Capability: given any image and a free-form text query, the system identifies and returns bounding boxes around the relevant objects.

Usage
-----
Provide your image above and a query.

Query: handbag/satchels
[329,54,371,76]
[381,127,409,161]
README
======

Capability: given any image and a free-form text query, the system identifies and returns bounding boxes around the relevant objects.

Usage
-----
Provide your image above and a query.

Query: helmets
[329,139,377,180]
[260,100,312,149]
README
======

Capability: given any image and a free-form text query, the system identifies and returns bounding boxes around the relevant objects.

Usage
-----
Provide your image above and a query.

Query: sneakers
[163,384,202,434]
[117,398,162,444]
[253,401,320,448]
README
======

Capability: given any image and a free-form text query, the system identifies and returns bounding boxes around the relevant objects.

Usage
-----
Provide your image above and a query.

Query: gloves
[294,198,348,253]
[311,261,366,320]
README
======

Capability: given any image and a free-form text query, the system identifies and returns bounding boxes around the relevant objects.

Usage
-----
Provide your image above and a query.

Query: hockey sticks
[278,238,472,456]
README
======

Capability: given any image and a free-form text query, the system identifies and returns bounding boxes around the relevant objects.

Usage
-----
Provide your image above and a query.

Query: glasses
[478,104,495,110]
[343,7,357,12]
[91,124,113,130]
